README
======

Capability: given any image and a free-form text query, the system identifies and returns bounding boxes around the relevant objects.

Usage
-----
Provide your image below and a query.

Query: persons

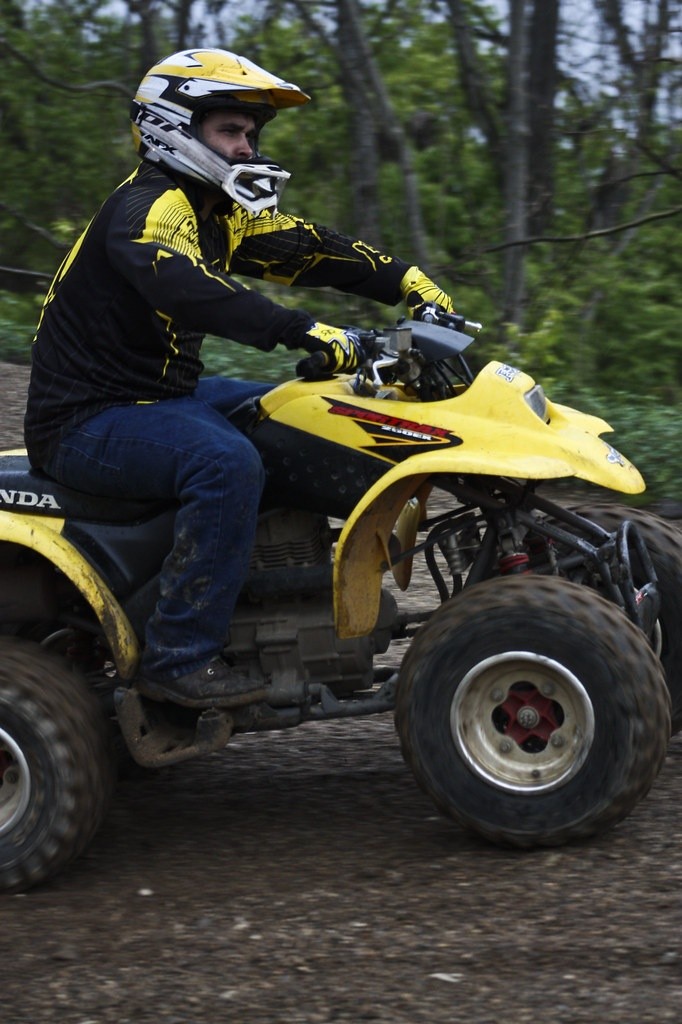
[23,47,455,707]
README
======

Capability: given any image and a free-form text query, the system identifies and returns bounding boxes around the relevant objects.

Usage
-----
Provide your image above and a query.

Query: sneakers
[136,655,268,709]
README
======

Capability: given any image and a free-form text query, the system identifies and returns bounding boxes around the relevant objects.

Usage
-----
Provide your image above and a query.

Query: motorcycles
[0,292,682,897]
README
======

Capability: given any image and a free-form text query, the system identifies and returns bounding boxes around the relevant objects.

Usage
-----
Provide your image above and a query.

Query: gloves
[284,308,359,377]
[399,264,454,318]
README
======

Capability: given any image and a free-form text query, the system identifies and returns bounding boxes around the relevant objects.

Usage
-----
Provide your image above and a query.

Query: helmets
[129,47,312,218]
